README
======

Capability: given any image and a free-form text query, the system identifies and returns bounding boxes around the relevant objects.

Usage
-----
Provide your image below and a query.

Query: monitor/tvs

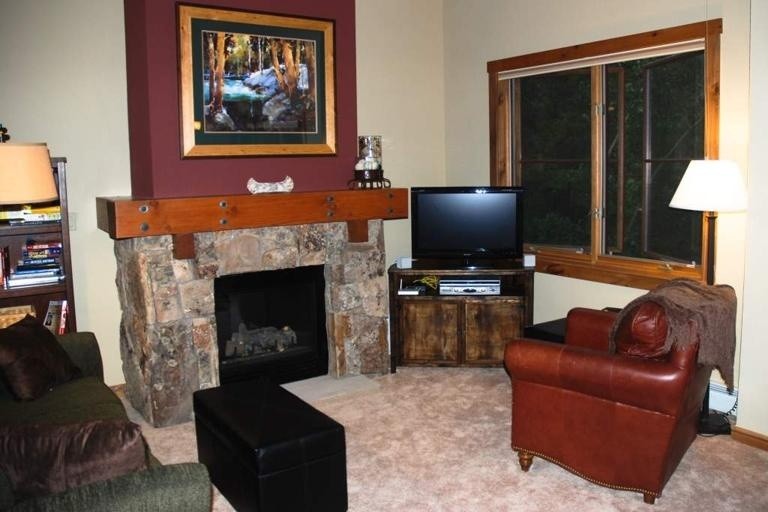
[409,185,524,270]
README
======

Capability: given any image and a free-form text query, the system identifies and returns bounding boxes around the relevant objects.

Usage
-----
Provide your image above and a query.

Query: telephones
[397,255,412,269]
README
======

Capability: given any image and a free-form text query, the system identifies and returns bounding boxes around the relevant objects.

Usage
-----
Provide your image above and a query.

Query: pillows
[0,417,149,495]
[1,314,83,402]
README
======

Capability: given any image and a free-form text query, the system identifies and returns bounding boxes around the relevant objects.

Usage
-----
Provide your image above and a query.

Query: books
[0,203,68,335]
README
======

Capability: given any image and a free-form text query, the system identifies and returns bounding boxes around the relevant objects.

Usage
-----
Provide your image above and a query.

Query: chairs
[504,284,736,504]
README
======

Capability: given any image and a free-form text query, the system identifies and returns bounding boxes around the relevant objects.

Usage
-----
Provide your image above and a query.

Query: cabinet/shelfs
[387,263,536,373]
[0,157,77,335]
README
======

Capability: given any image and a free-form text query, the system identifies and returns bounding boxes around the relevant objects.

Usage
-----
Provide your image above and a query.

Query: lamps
[668,159,750,435]
[0,143,59,205]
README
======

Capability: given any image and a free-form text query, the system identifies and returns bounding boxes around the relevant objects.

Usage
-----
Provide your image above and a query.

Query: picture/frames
[175,2,338,159]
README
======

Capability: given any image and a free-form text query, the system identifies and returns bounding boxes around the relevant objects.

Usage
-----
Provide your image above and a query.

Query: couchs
[0,332,213,512]
